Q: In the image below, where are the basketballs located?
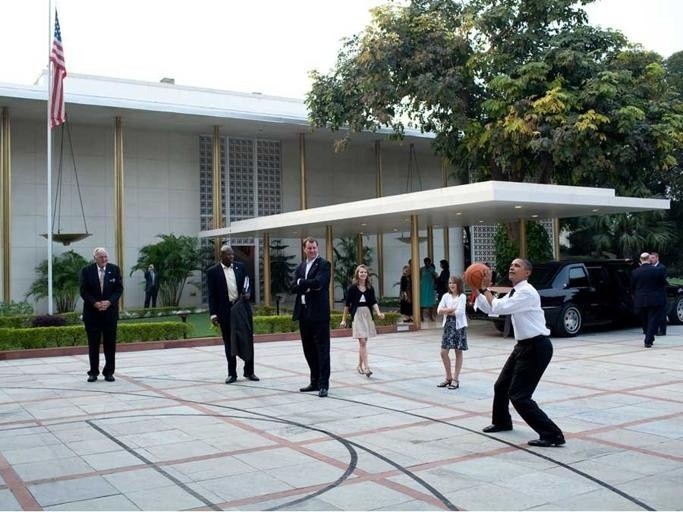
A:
[463,263,489,288]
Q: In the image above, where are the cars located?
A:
[466,259,683,336]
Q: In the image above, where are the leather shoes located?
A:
[527,434,566,447]
[86,375,98,382]
[318,388,329,397]
[300,383,319,392]
[482,423,514,433]
[224,374,238,384]
[243,372,260,382]
[105,374,115,381]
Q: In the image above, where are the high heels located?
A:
[449,379,459,389]
[437,377,452,388]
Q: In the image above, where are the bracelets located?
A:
[482,288,491,295]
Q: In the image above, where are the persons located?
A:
[631,252,666,347]
[288,239,331,397]
[144,264,159,307]
[207,245,260,383]
[79,247,124,382]
[340,265,385,377]
[470,257,566,447]
[400,258,450,323]
[436,275,468,389]
[639,252,666,336]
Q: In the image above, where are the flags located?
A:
[50,8,66,128]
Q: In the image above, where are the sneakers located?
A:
[645,341,654,348]
[356,365,374,378]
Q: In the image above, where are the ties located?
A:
[99,266,106,294]
[503,289,516,338]
